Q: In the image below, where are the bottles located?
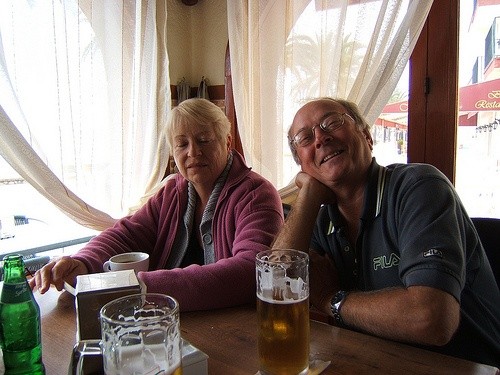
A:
[0,254,46,375]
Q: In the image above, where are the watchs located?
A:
[329,289,351,329]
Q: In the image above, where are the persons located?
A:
[28,96,286,315]
[268,96,500,369]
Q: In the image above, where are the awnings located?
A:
[375,78,499,132]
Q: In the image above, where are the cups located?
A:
[100,293,182,375]
[256,249,310,375]
[103,252,149,272]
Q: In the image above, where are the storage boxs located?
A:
[74,268,141,375]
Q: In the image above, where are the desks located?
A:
[26,271,500,375]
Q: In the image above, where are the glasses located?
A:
[289,112,355,147]
